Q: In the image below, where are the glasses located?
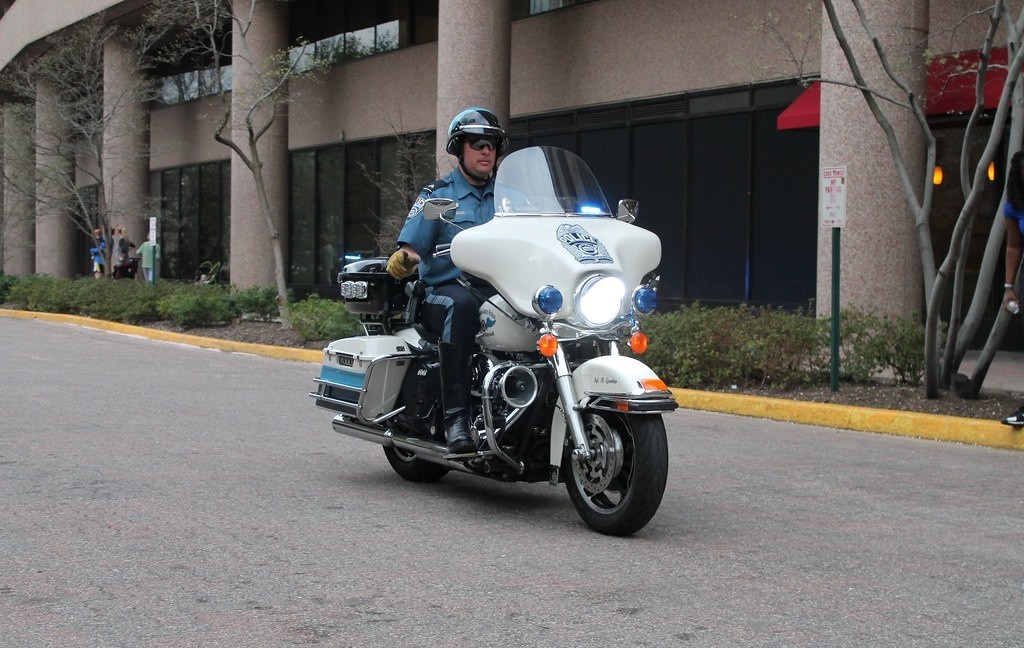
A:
[463,137,497,151]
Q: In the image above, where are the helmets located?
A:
[446,106,509,156]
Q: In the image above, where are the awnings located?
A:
[776,45,1024,130]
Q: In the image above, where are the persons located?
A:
[135,233,162,282]
[387,107,537,458]
[90,224,137,279]
[1002,152,1024,425]
[201,274,207,282]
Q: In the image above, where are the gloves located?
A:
[387,251,421,280]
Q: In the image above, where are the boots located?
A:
[437,340,478,453]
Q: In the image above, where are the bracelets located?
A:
[1005,284,1012,288]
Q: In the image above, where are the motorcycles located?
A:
[309,146,677,537]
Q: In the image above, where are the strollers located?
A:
[193,262,222,285]
[113,258,139,281]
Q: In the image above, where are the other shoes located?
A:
[1001,406,1024,425]
